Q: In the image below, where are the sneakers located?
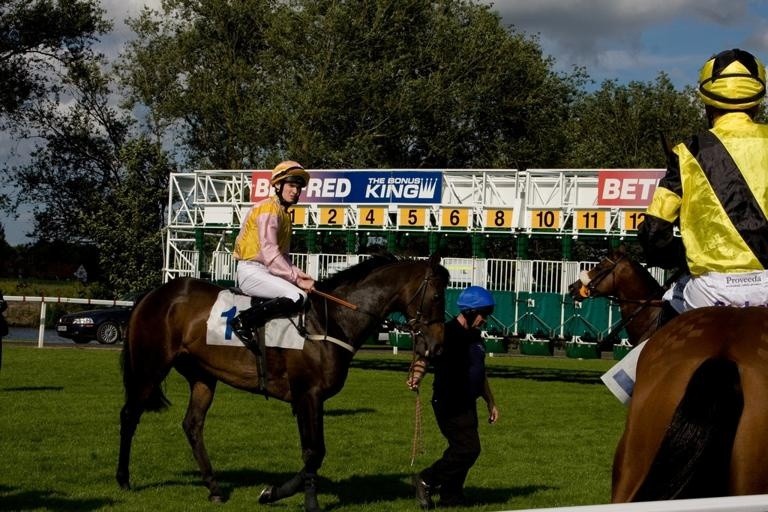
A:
[414,472,432,510]
[439,492,478,507]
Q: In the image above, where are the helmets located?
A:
[694,48,766,110]
[456,285,496,313]
[269,160,311,188]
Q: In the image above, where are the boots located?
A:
[228,297,298,356]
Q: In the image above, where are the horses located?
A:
[611,305,768,503]
[568,242,666,350]
[115,246,450,512]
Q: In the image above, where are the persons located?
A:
[405,284,498,508]
[225,159,314,353]
[635,47,767,330]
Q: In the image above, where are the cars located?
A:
[58,289,150,346]
